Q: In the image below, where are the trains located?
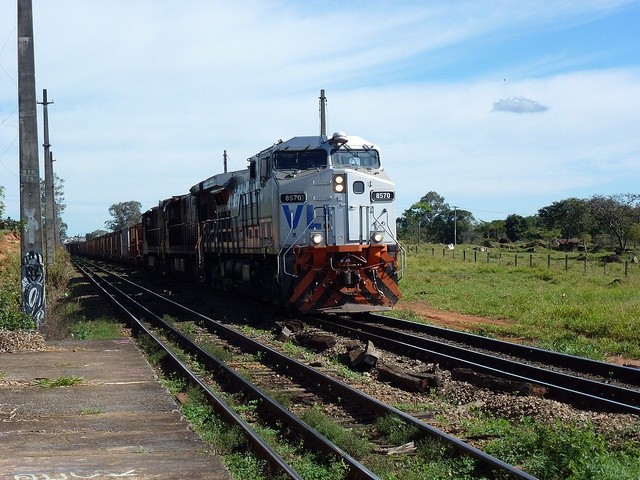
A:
[65,132,406,322]
